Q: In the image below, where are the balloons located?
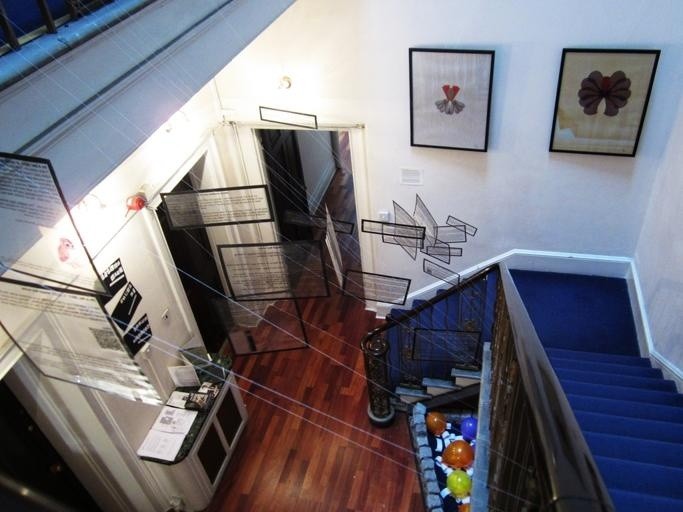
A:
[460,416,478,442]
[441,439,474,469]
[426,411,446,435]
[446,468,472,499]
[458,502,471,512]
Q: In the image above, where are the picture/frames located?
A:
[408,46,495,152]
[548,47,661,158]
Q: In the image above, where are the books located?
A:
[167,352,201,388]
[184,382,220,412]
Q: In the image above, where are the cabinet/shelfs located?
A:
[137,370,248,511]
